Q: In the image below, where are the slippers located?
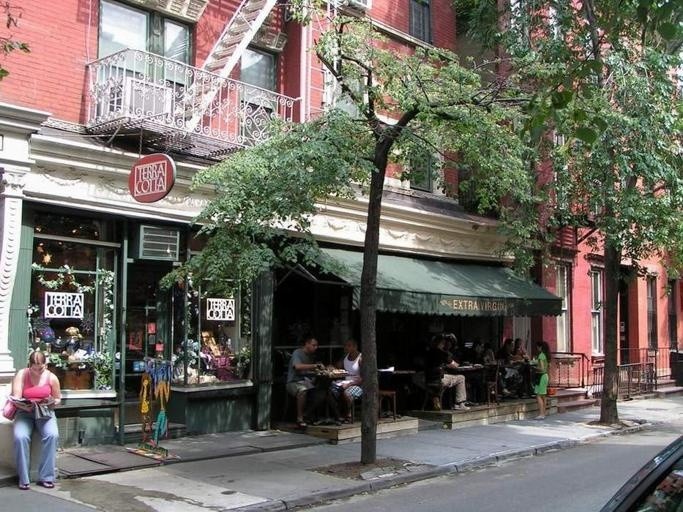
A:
[297,419,307,427]
[18,482,30,489]
[37,481,54,488]
[337,415,351,425]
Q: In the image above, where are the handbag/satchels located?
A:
[3,400,17,419]
[534,373,543,385]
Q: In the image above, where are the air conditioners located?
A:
[108,77,173,123]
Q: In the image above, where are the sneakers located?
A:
[433,397,440,411]
[454,402,471,411]
[534,415,546,420]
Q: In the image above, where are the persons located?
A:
[326,338,363,426]
[284,337,326,428]
[528,341,552,419]
[9,350,62,490]
[424,331,533,412]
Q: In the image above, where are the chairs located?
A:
[283,360,533,423]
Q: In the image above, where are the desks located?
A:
[46,362,93,389]
